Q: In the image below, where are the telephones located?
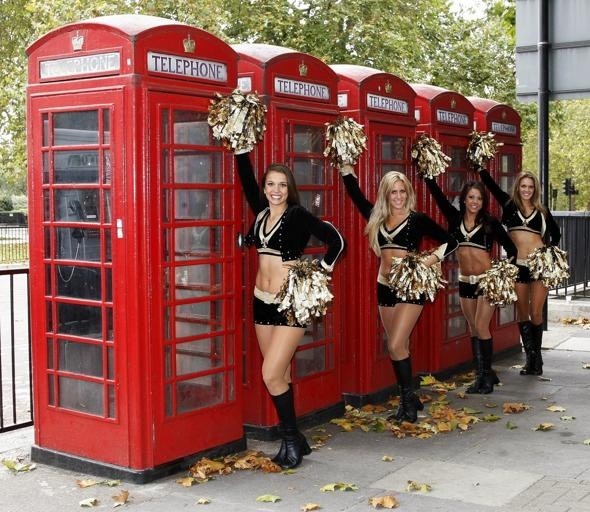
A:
[56,189,111,268]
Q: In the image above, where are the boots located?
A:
[387,353,425,426]
[517,320,545,376]
[476,337,500,394]
[465,335,483,394]
[271,383,312,470]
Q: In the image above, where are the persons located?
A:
[419,172,517,394]
[231,146,346,469]
[339,163,462,424]
[473,164,572,376]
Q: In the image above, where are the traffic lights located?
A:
[553,188,557,198]
[562,178,571,195]
